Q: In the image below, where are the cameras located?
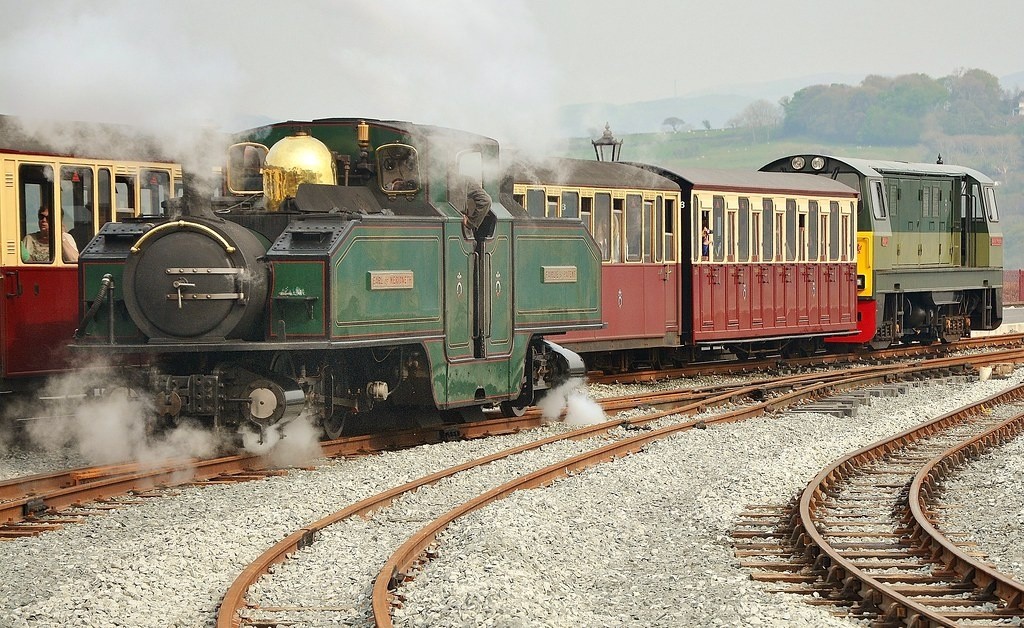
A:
[707,230,713,234]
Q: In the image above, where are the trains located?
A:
[0,118,1004,456]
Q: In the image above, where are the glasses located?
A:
[38,213,50,220]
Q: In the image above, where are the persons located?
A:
[23,203,80,264]
[594,210,631,264]
[455,165,494,233]
[699,224,716,265]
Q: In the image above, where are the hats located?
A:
[798,217,804,225]
